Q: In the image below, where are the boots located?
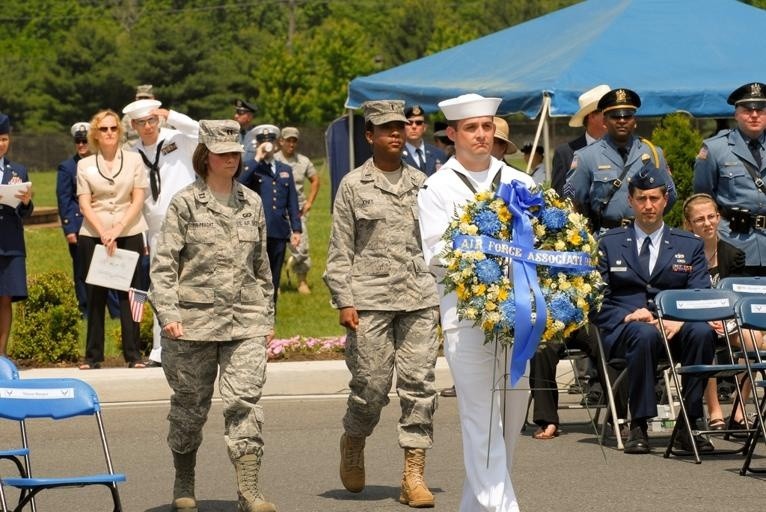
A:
[232,454,276,512]
[172,449,198,512]
[340,433,365,492]
[296,273,311,294]
[400,448,435,507]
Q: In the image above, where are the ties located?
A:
[267,162,272,171]
[639,237,650,277]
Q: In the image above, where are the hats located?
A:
[0,85,299,155]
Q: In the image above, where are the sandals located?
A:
[129,361,145,368]
[80,362,100,369]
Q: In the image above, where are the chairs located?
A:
[2,357,126,511]
[594,274,765,477]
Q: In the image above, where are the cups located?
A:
[263,143,273,153]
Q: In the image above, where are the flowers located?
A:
[443,179,605,346]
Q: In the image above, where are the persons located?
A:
[432,125,457,154]
[692,80,766,268]
[681,192,765,432]
[56,122,121,321]
[121,84,173,149]
[552,84,608,198]
[519,145,546,187]
[76,107,151,369]
[277,128,321,296]
[147,119,278,512]
[231,100,256,139]
[533,318,635,441]
[0,111,34,353]
[418,91,537,511]
[122,99,201,367]
[566,90,677,229]
[589,167,717,456]
[321,96,439,507]
[241,125,302,306]
[490,117,524,173]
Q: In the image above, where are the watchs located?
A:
[397,105,446,179]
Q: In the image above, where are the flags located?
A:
[127,287,148,323]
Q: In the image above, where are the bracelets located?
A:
[117,221,125,227]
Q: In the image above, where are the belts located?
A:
[718,204,766,231]
[600,218,634,229]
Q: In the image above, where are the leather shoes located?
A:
[441,384,456,397]
[568,375,757,454]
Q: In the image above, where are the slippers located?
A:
[532,424,558,439]
